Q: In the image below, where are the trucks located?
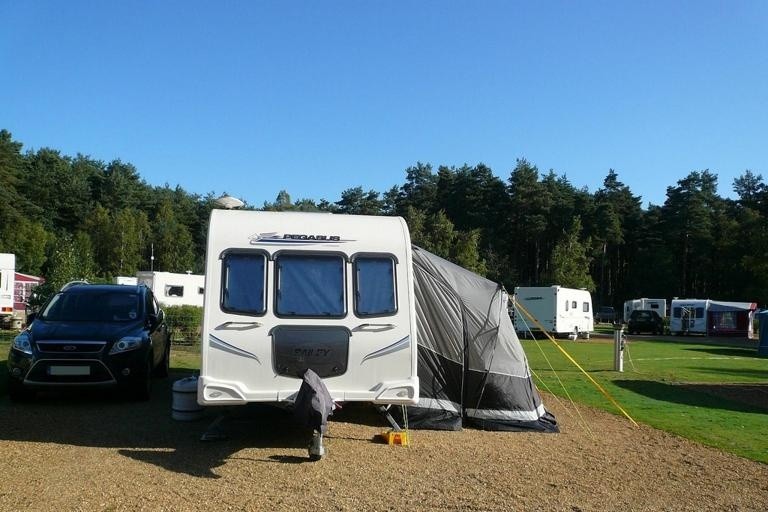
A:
[599,307,618,322]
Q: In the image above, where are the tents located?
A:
[375,243,562,435]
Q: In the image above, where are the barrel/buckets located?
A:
[10,317,23,329]
[173,376,206,421]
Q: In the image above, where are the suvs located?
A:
[627,310,663,336]
[7,280,172,400]
